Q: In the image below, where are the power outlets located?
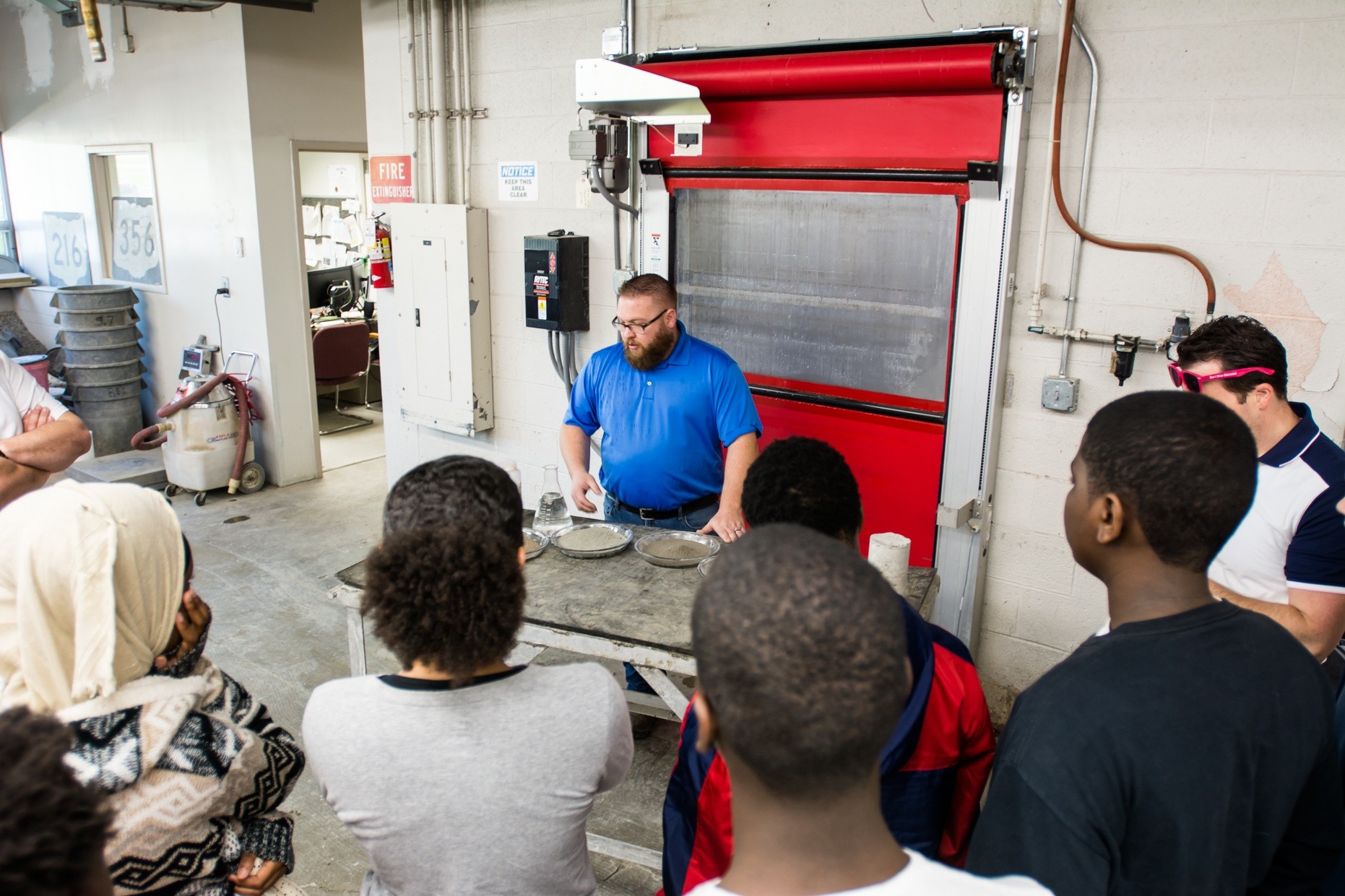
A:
[223,278,230,298]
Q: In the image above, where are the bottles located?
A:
[505,459,522,500]
[532,464,573,538]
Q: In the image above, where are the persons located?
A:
[1,349,306,896]
[300,457,631,895]
[1178,315,1345,702]
[561,273,762,542]
[964,390,1345,896]
[682,526,1057,896]
[661,435,990,896]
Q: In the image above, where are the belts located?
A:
[607,490,719,520]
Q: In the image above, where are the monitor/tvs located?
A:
[309,258,370,320]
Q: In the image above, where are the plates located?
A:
[634,531,720,567]
[523,528,550,561]
[550,523,634,559]
[695,556,718,577]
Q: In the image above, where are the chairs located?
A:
[314,322,373,435]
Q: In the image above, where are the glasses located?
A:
[612,308,667,334]
[1167,361,1276,394]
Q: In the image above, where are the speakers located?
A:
[363,299,374,320]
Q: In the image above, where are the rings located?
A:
[735,529,741,533]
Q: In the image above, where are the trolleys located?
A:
[163,350,267,507]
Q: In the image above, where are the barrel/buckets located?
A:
[49,285,148,458]
[11,354,50,391]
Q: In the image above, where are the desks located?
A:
[309,309,381,404]
[326,508,943,870]
